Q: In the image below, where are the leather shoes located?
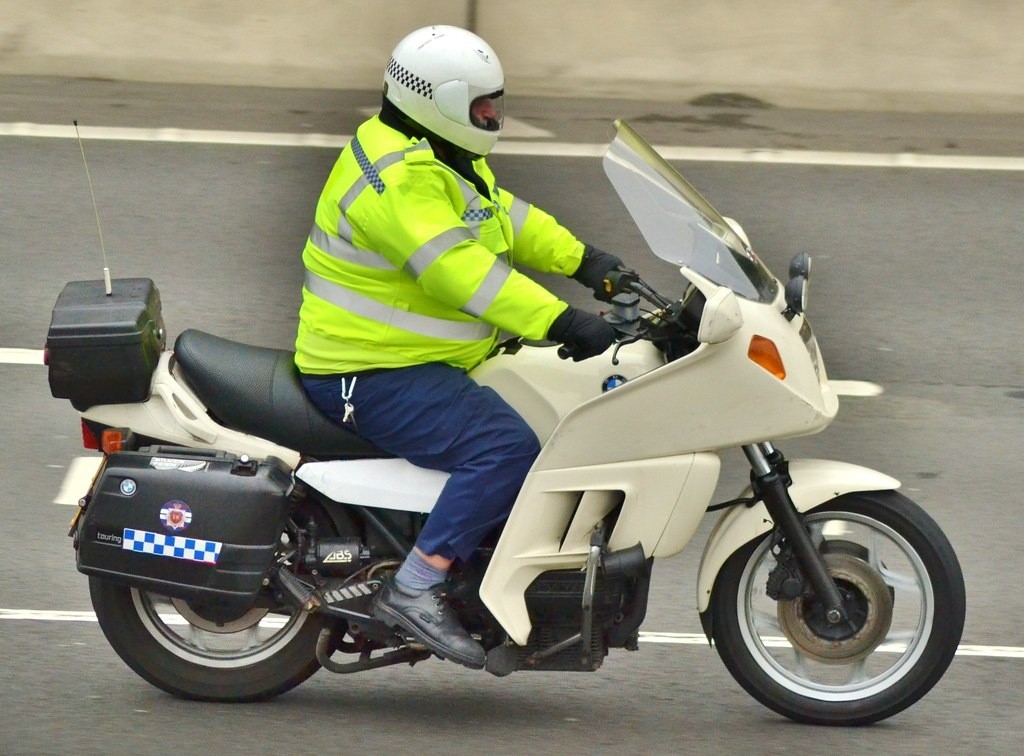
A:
[367,577,488,669]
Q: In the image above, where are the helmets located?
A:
[382,24,509,162]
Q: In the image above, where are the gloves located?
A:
[577,244,628,303]
[547,305,618,362]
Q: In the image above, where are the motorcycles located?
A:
[42,118,968,726]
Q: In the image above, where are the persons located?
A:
[295,24,628,669]
